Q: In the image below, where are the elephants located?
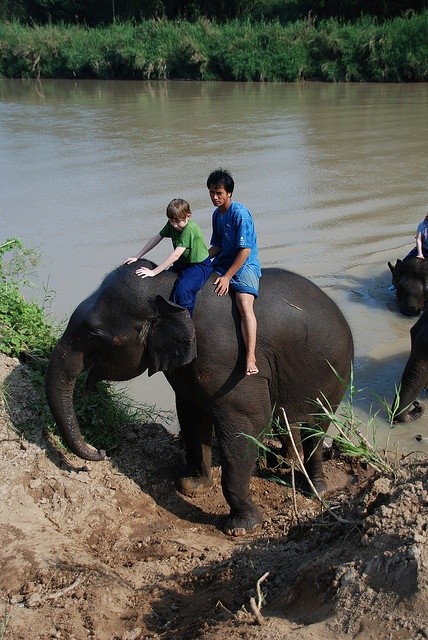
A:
[387,245,427,317]
[390,297,427,422]
[44,257,353,537]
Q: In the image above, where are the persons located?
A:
[415,212,427,261]
[121,198,214,310]
[207,166,262,375]
